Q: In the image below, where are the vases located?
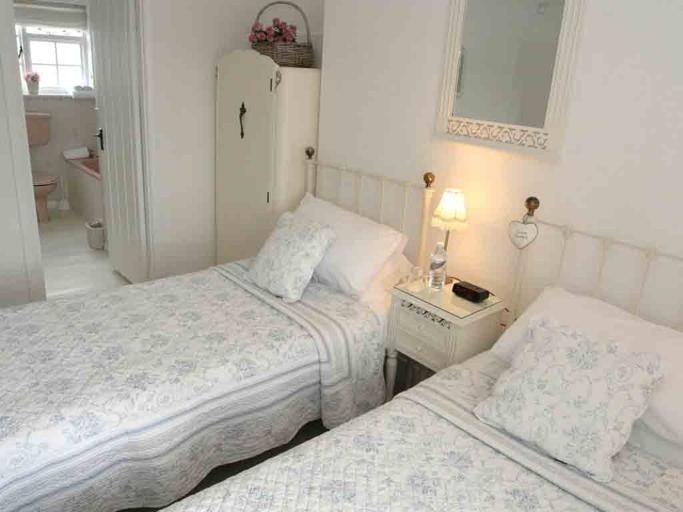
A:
[26,81,38,95]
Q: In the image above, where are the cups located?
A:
[407,265,427,293]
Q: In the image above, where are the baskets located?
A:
[252,2,314,65]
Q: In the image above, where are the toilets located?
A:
[24,112,57,224]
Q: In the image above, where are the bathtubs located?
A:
[66,157,100,179]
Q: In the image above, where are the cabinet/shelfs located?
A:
[212,48,318,264]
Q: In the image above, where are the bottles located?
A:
[430,242,448,291]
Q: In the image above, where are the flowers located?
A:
[23,72,40,87]
[248,17,297,49]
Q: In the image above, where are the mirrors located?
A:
[434,1,584,155]
